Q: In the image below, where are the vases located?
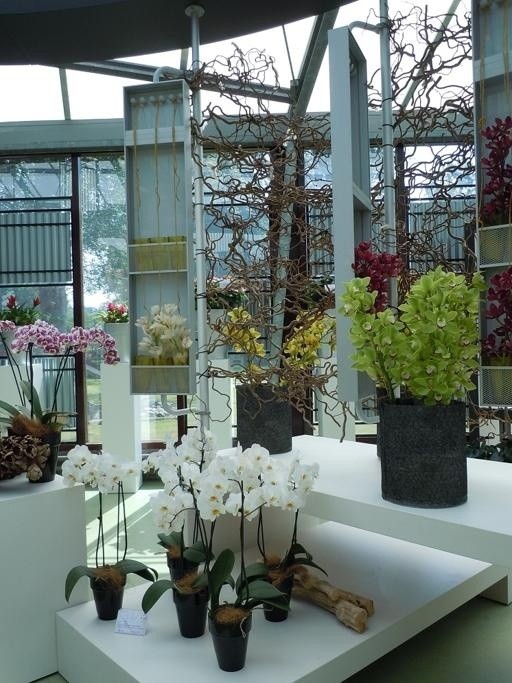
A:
[236,383,292,454]
[91,573,292,672]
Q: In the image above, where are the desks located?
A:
[53,433,512,682]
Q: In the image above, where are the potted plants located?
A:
[338,264,487,509]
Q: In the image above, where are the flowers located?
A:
[219,306,338,386]
[0,295,121,481]
[140,432,329,611]
[61,445,159,602]
[134,304,194,365]
